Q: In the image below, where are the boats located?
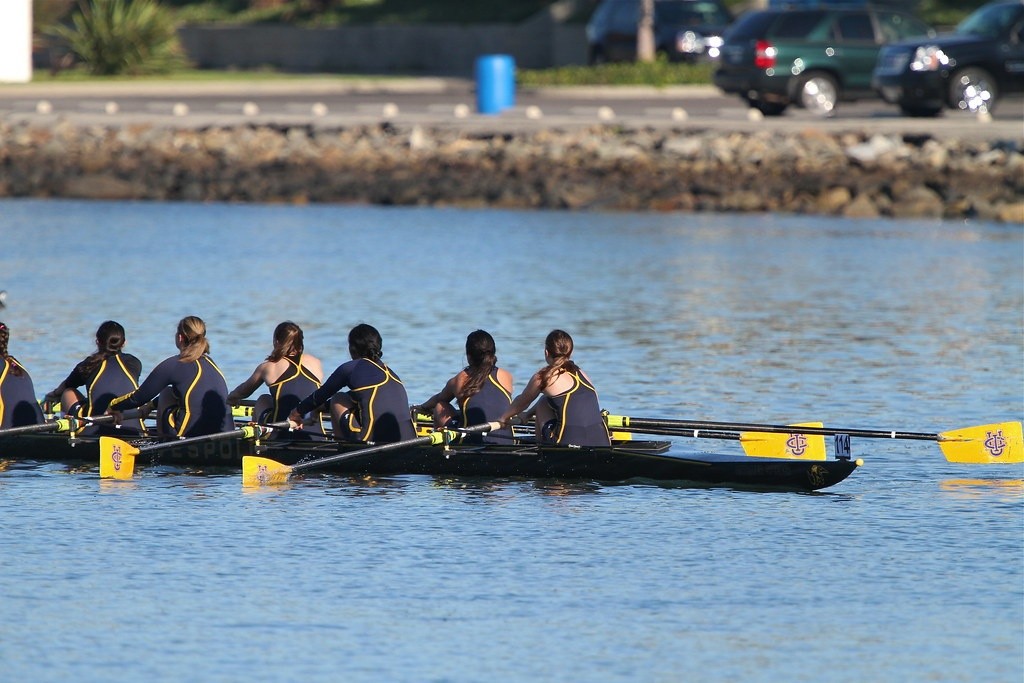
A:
[0,404,866,492]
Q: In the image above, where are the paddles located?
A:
[0,387,1024,496]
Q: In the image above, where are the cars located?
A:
[710,0,943,121]
[869,0,1024,116]
[582,1,739,66]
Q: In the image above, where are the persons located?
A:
[285,323,418,446]
[407,328,519,445]
[493,329,611,448]
[225,320,328,443]
[0,322,48,432]
[38,320,148,439]
[102,316,235,444]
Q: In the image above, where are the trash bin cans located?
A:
[473,54,515,113]
